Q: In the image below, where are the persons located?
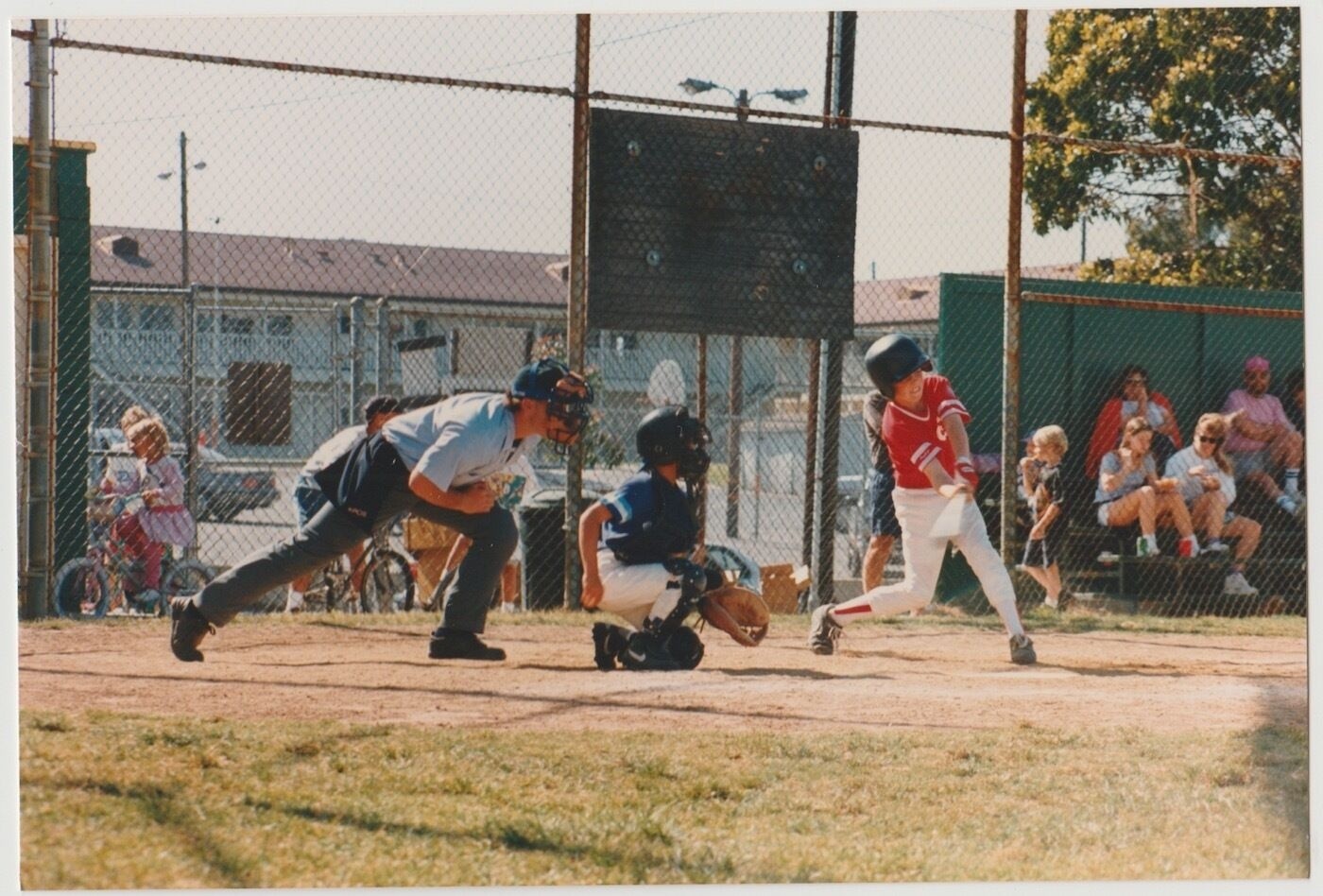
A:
[96,405,196,615]
[808,335,1035,664]
[171,358,593,665]
[1018,425,1079,612]
[576,406,728,668]
[439,453,542,612]
[287,396,404,613]
[862,389,929,616]
[1085,357,1307,594]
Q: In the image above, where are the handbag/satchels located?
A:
[313,436,400,536]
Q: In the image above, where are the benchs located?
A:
[985,472,1307,616]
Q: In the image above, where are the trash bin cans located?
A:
[514,486,604,613]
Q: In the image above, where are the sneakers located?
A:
[809,604,844,655]
[592,624,623,671]
[429,631,507,661]
[1009,634,1036,664]
[169,596,216,662]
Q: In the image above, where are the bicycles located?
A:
[303,509,415,613]
[49,491,216,621]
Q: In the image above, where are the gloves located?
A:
[954,458,978,494]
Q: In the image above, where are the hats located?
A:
[1020,430,1037,442]
[1245,355,1270,371]
[512,356,571,402]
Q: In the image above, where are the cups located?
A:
[1179,540,1191,558]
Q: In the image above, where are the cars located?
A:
[167,442,277,529]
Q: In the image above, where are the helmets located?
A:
[865,333,933,400]
[636,405,704,472]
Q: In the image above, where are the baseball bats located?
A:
[930,492,968,544]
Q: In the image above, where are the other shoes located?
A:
[1209,539,1228,553]
[1199,536,1220,556]
[1040,603,1053,610]
[1148,551,1174,560]
[106,600,155,618]
[1058,590,1074,611]
[1285,486,1302,503]
[1180,536,1210,557]
[1224,574,1258,596]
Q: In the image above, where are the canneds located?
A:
[1136,537,1149,557]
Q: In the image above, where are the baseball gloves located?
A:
[697,585,770,647]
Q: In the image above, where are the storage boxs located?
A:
[406,515,461,551]
[726,564,799,614]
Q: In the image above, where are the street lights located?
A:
[156,128,207,556]
[677,77,809,540]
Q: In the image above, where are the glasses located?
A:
[1248,373,1269,380]
[1196,432,1218,444]
